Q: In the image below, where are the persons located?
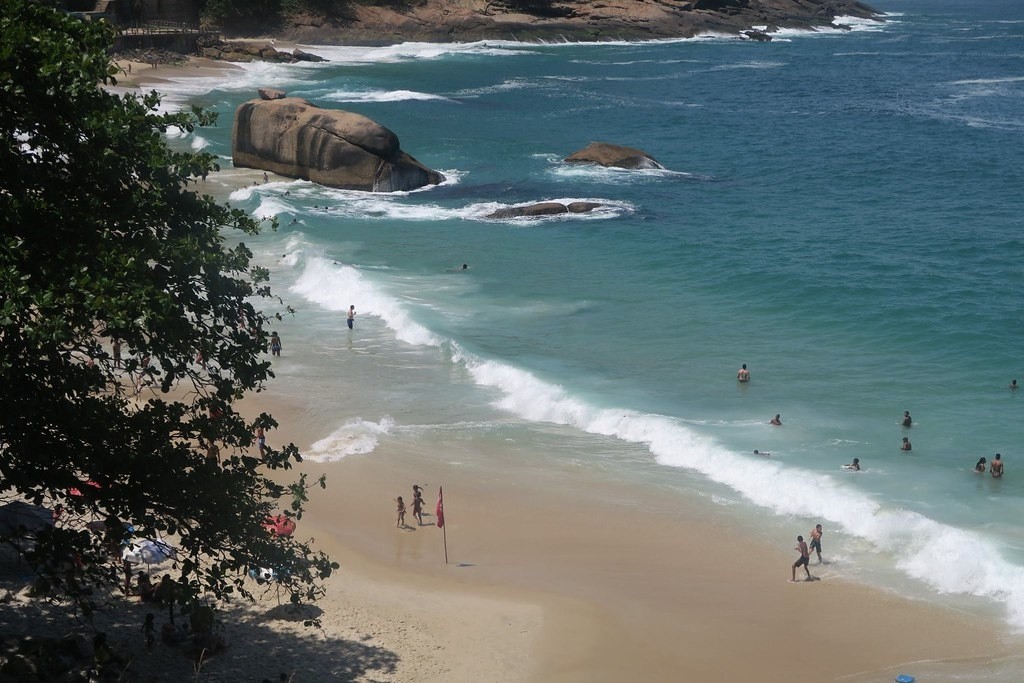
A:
[902,410,912,428]
[807,524,823,564]
[1009,379,1019,391]
[753,449,770,456]
[410,485,425,525]
[393,496,407,527]
[989,453,1004,479]
[346,305,356,330]
[736,363,750,383]
[768,414,782,425]
[242,171,470,272]
[976,457,986,473]
[900,437,912,451]
[83,304,305,649]
[849,458,860,472]
[787,535,812,583]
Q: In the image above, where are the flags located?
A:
[436,486,444,528]
